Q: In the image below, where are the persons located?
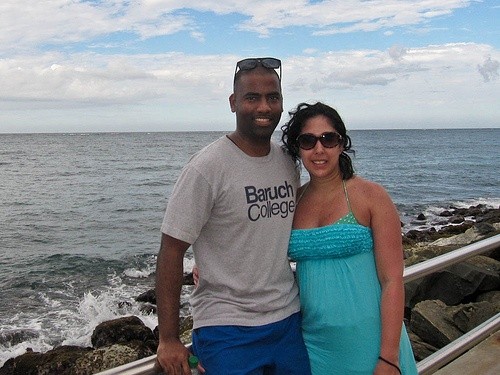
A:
[192,101,418,375]
[157,62,311,375]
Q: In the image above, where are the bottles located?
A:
[181,355,201,375]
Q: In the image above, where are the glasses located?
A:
[233,58,282,87]
[294,131,345,150]
